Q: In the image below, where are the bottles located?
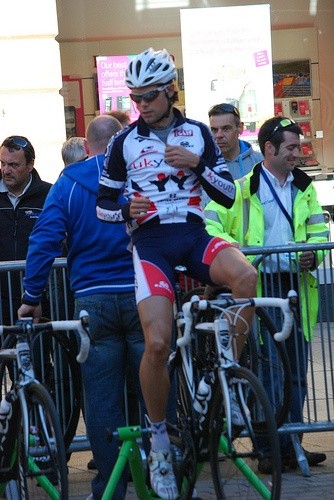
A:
[192,375,211,414]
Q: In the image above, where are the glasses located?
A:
[129,84,170,103]
[222,104,240,119]
[268,118,296,141]
[5,137,35,159]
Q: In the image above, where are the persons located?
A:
[0,135,80,488]
[204,102,264,183]
[16,113,181,500]
[95,48,262,500]
[60,135,90,169]
[202,114,333,473]
[104,111,131,128]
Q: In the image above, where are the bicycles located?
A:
[144,261,299,500]
[0,310,92,497]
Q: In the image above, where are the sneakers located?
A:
[146,449,180,500]
[194,376,251,426]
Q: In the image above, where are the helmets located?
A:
[124,47,176,89]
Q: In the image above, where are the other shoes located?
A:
[281,442,326,468]
[254,445,285,474]
